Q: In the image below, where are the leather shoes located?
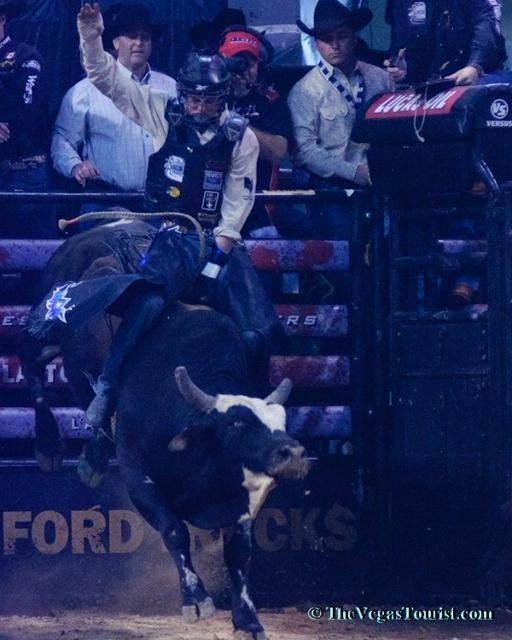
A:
[83,374,121,431]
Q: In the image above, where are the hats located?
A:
[101,6,166,51]
[295,0,373,37]
[220,30,265,60]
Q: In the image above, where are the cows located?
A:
[11,204,313,639]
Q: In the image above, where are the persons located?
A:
[0,0,510,306]
[76,2,271,442]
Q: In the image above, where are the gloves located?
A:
[191,243,229,304]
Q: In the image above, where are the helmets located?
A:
[175,47,232,124]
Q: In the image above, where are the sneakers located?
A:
[441,291,474,320]
